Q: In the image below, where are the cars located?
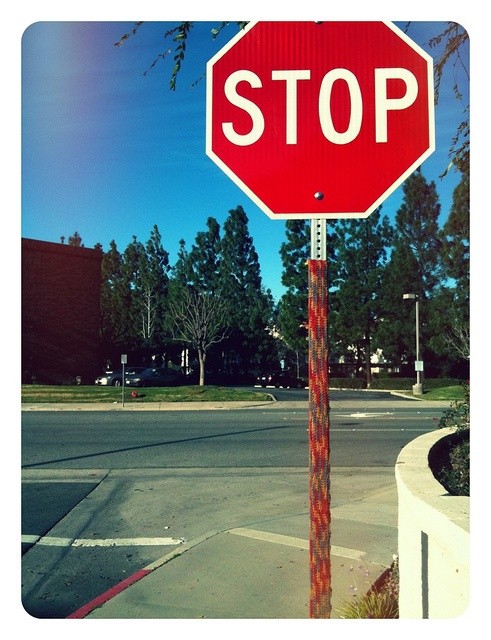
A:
[126,368,186,387]
[257,371,305,389]
[95,367,146,386]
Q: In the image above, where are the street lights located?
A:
[403,294,421,383]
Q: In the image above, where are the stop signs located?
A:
[205,21,435,220]
